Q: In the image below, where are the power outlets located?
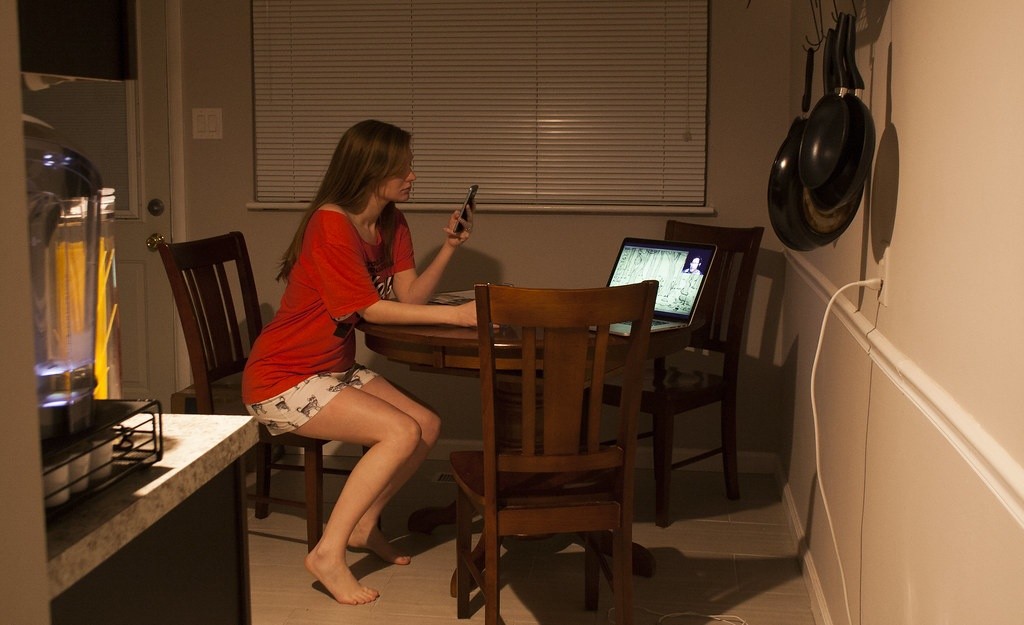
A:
[874,241,890,306]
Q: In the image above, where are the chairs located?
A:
[156,231,382,558]
[445,279,660,625]
[582,220,765,528]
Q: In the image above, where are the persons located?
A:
[242,119,500,604]
[684,255,703,275]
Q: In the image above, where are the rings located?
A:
[465,225,472,231]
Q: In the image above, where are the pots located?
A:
[766,12,875,250]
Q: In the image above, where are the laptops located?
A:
[589,238,718,337]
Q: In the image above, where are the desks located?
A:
[353,289,706,599]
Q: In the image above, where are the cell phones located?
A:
[453,184,479,233]
